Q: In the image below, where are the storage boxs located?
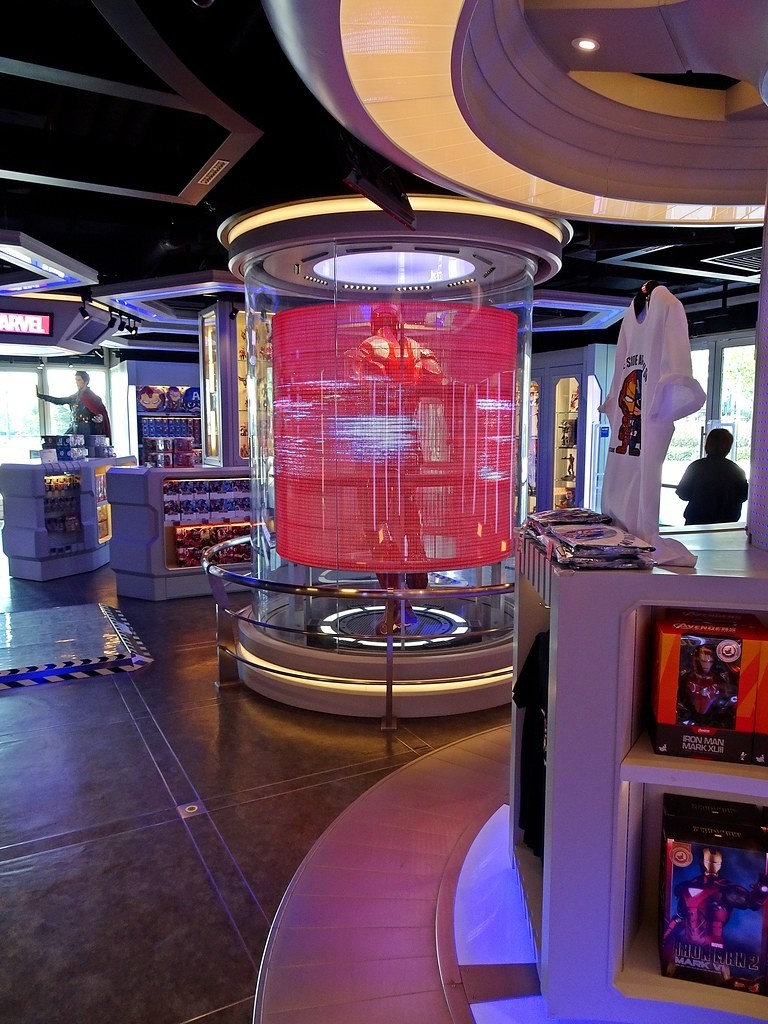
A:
[41,435,115,459]
[142,417,201,468]
[163,479,253,567]
[650,610,768,768]
[659,793,768,997]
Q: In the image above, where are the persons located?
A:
[346,302,471,636]
[36,371,112,445]
[562,454,574,475]
[676,429,749,526]
[677,645,739,729]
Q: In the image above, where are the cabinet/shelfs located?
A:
[0,455,138,581]
[106,466,252,601]
[554,410,579,483]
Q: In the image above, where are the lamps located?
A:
[78,296,93,320]
[108,306,142,336]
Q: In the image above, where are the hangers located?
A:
[632,278,670,314]
